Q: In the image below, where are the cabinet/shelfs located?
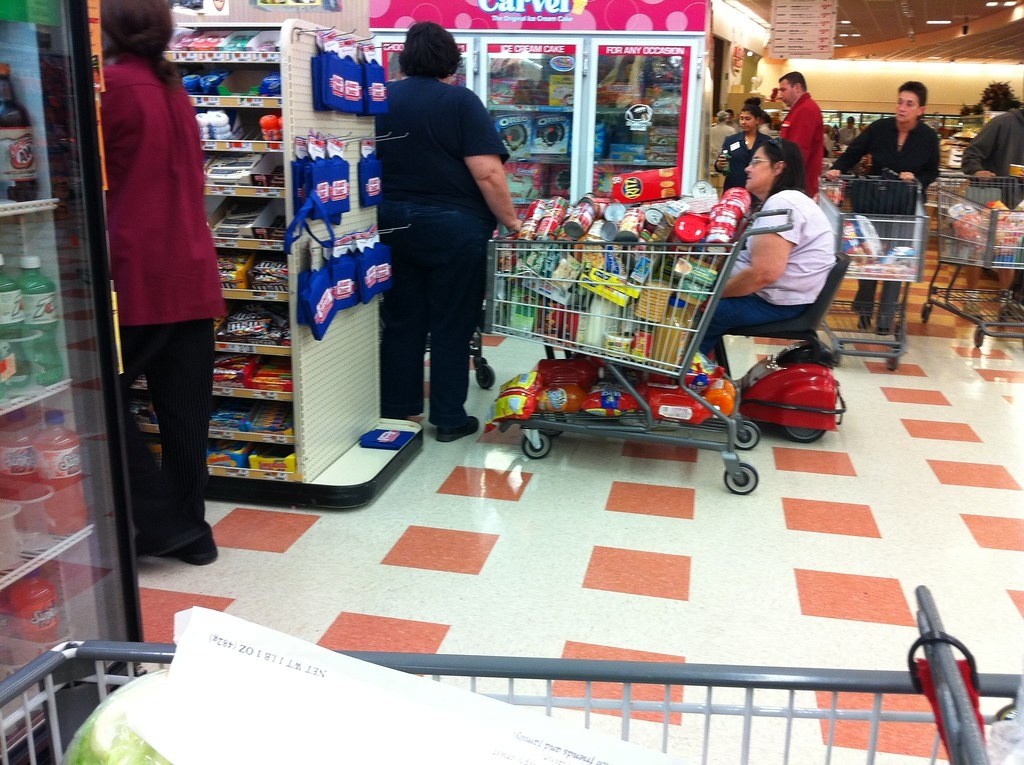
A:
[164,17,424,512]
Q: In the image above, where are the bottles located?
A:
[648,297,691,372]
[0,253,65,400]
[0,62,39,201]
[981,202,1003,246]
[722,150,731,176]
[534,358,604,414]
[0,568,61,648]
[648,376,735,423]
[0,408,88,537]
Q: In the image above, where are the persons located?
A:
[378,21,523,443]
[697,138,837,378]
[710,109,739,163]
[778,71,825,203]
[828,81,939,334]
[837,115,860,144]
[94,0,227,566]
[961,107,1024,304]
[714,97,774,196]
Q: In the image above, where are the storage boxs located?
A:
[648,212,675,251]
[167,25,297,478]
[589,269,642,299]
[489,77,682,219]
[521,278,577,306]
[630,255,662,284]
[578,273,629,308]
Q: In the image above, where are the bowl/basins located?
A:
[604,331,635,358]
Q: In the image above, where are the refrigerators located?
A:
[0,1,142,765]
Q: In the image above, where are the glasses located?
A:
[748,159,773,166]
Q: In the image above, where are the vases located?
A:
[990,102,1009,111]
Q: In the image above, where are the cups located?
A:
[1,483,56,572]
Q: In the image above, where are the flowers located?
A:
[980,82,1020,107]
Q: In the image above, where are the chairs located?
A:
[713,250,851,379]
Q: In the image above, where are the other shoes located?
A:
[858,315,870,329]
[877,327,890,335]
[438,415,478,441]
[135,530,218,564]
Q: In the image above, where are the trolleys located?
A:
[471,206,795,498]
[922,172,1024,347]
[820,166,930,371]
[0,580,1024,765]
